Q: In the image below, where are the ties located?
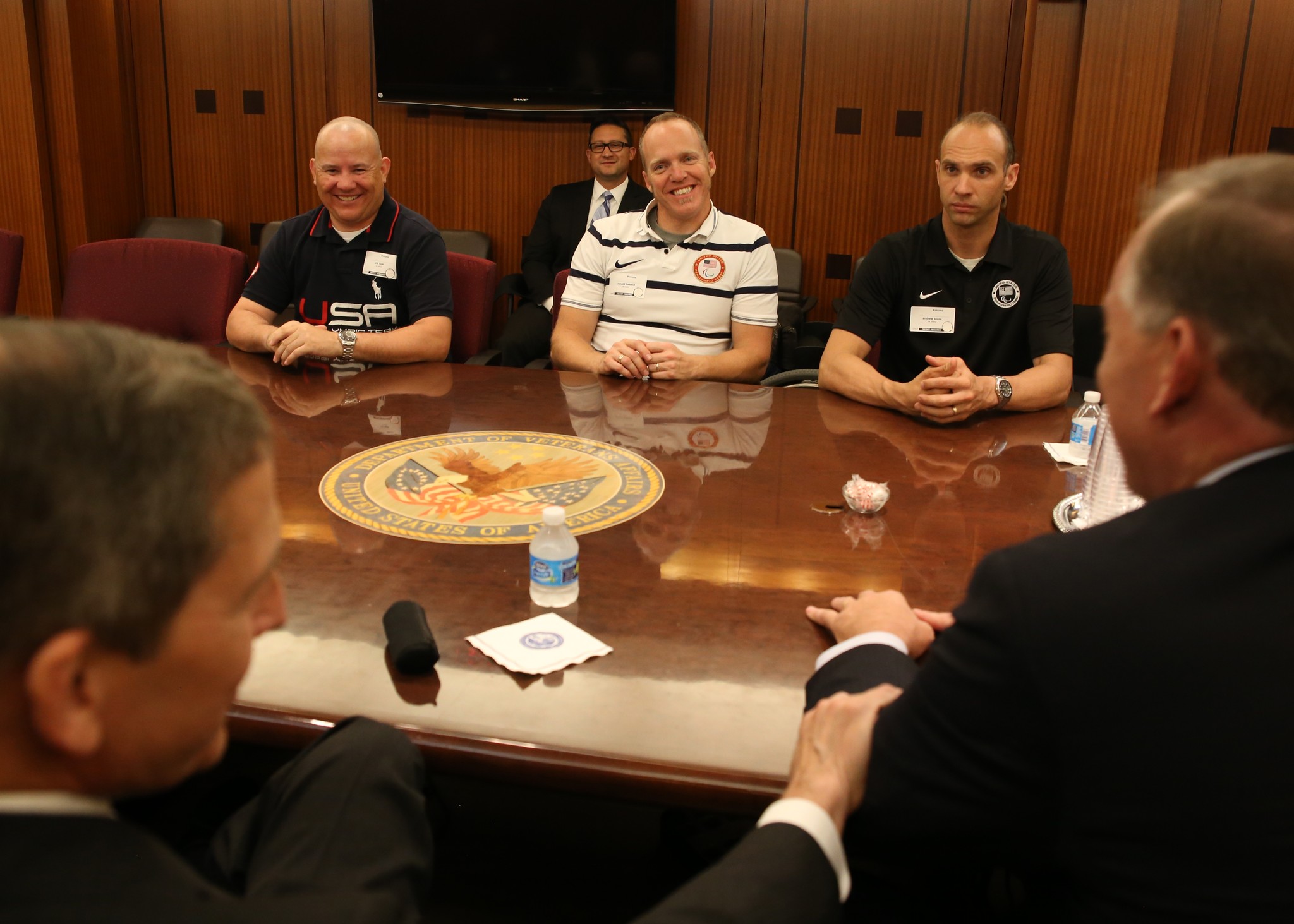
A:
[591,192,613,226]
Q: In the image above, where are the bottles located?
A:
[529,506,581,608]
[1067,391,1101,461]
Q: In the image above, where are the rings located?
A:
[618,354,625,363]
[951,405,958,415]
[656,363,659,371]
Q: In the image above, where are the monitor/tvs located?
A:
[370,0,679,122]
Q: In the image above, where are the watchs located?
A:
[985,375,1012,410]
[338,330,357,364]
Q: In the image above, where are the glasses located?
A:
[589,141,630,152]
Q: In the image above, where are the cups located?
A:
[1080,404,1147,529]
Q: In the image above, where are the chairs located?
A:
[134,215,225,244]
[60,238,246,348]
[1070,303,1105,391]
[444,251,504,368]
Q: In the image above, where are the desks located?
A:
[167,347,1141,815]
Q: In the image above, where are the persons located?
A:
[1,319,906,924]
[226,117,454,364]
[807,152,1294,923]
[497,115,654,367]
[549,111,779,383]
[816,111,1074,424]
